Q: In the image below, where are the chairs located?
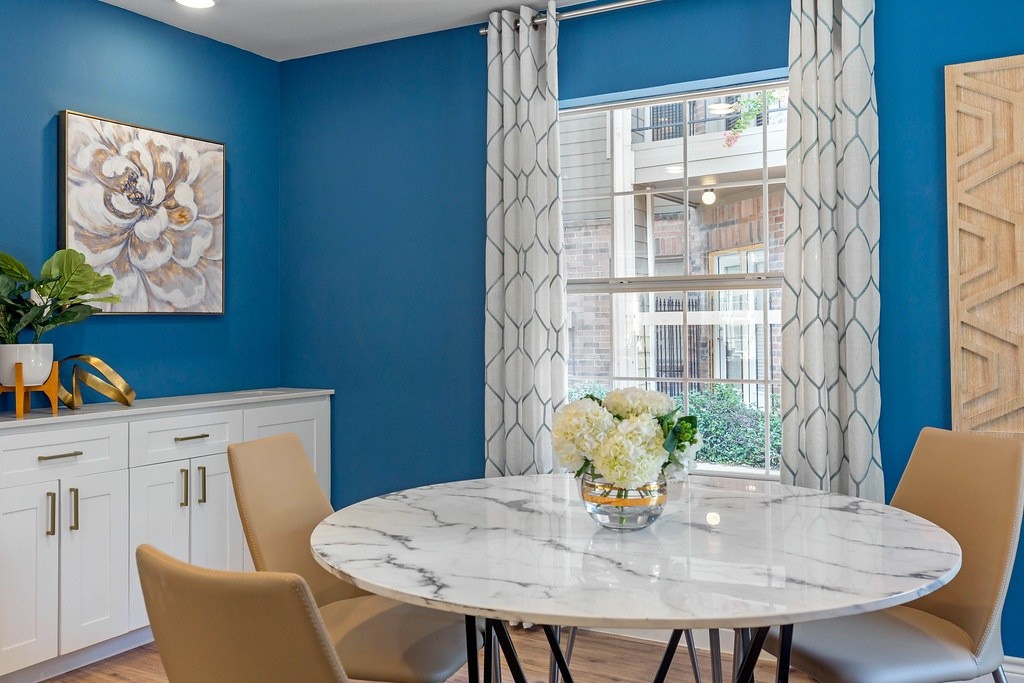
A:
[229,434,504,683]
[134,544,348,683]
[739,426,1024,683]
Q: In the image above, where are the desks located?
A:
[311,471,962,683]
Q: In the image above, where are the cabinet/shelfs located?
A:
[0,386,336,683]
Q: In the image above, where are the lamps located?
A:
[702,189,717,205]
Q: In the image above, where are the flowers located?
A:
[552,386,704,509]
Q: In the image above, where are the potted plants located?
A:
[0,248,123,388]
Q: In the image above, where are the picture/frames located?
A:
[57,109,226,318]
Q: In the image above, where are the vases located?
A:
[579,467,668,533]
